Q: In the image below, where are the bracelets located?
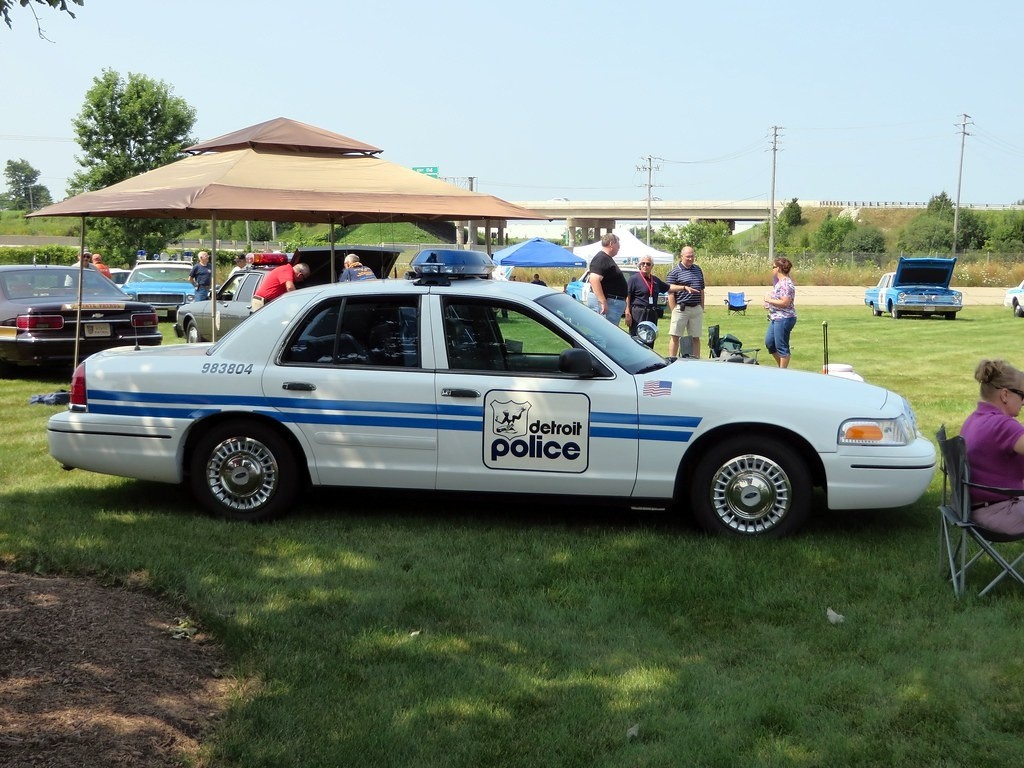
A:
[684,286,685,290]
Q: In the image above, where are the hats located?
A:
[78,246,92,255]
[233,253,246,262]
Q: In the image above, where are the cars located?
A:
[174,246,407,344]
[865,256,962,320]
[1003,280,1024,317]
[48,249,935,539]
[565,264,667,318]
[106,250,196,321]
[0,252,162,380]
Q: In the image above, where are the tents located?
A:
[574,228,673,270]
[25,116,566,373]
[494,237,586,268]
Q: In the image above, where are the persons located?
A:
[92,254,111,277]
[763,258,797,367]
[531,274,546,286]
[188,252,212,302]
[228,254,251,286]
[66,247,96,286]
[338,254,376,282]
[625,256,700,349]
[587,233,629,326]
[254,263,310,304]
[961,359,1024,535]
[666,246,706,358]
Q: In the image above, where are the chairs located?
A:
[936,423,1024,603]
[707,324,760,364]
[447,318,487,370]
[723,291,752,316]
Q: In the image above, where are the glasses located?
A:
[773,266,778,269]
[299,270,306,280]
[83,255,91,259]
[995,386,1024,400]
[641,262,651,266]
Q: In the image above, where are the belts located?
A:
[685,303,697,307]
[970,499,1006,510]
[635,305,656,308]
[253,296,268,302]
[608,295,625,300]
